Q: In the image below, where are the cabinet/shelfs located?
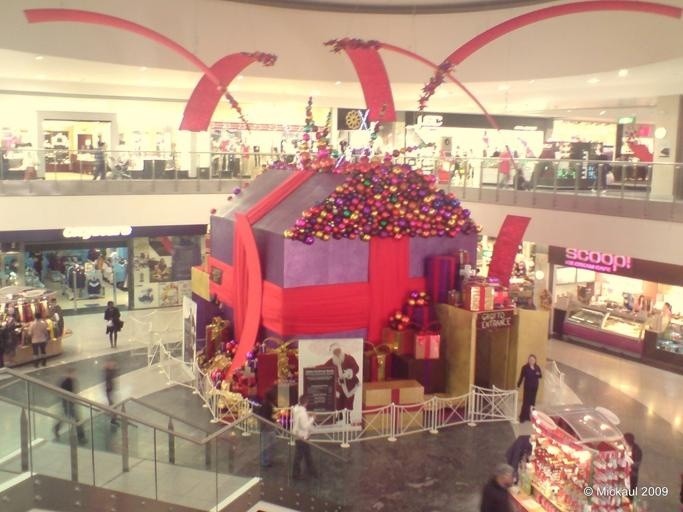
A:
[562,306,648,360]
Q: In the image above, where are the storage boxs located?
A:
[415,330,440,360]
[382,328,415,356]
[426,250,508,312]
[363,379,424,431]
[363,350,392,382]
[406,305,432,330]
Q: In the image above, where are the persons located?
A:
[515,354,542,424]
[498,144,512,189]
[31,251,44,283]
[508,434,532,470]
[27,312,48,368]
[103,301,124,349]
[621,432,642,503]
[51,298,62,317]
[479,463,517,512]
[290,394,318,478]
[256,386,283,467]
[92,141,107,181]
[629,295,644,317]
[52,364,89,445]
[103,357,121,425]
[323,343,360,425]
[657,302,672,340]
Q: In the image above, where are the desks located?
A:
[508,487,545,512]
[3,337,60,367]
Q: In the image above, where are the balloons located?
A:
[211,338,255,382]
[387,288,432,331]
[209,94,484,245]
